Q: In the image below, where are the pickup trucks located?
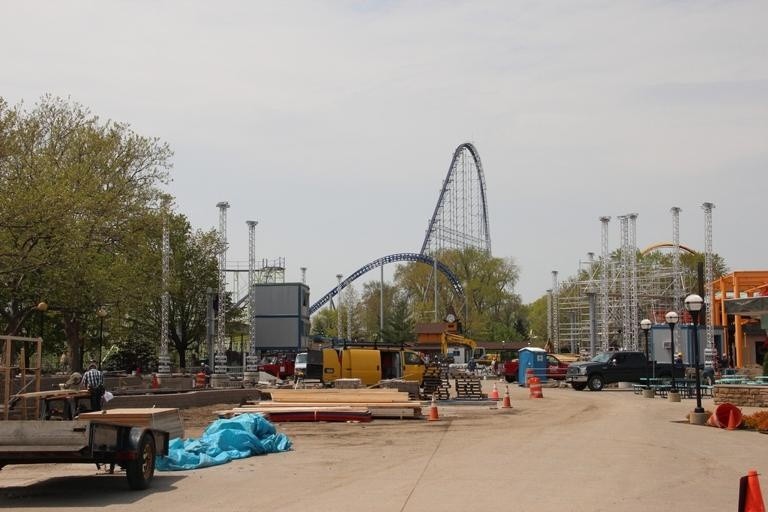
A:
[504,355,569,383]
[566,352,687,391]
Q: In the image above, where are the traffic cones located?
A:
[492,383,513,408]
[427,395,440,421]
[743,469,765,512]
[705,403,743,430]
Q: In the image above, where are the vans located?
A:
[322,347,434,387]
[294,353,307,378]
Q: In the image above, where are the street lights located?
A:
[640,312,679,393]
[684,294,704,413]
[38,302,47,363]
[96,308,107,370]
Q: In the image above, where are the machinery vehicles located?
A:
[441,330,500,369]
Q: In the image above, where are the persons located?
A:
[82,365,104,411]
[490,357,496,375]
[675,353,683,364]
[701,367,714,386]
[466,356,475,373]
[721,353,729,369]
[200,362,213,390]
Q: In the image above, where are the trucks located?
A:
[247,356,294,380]
